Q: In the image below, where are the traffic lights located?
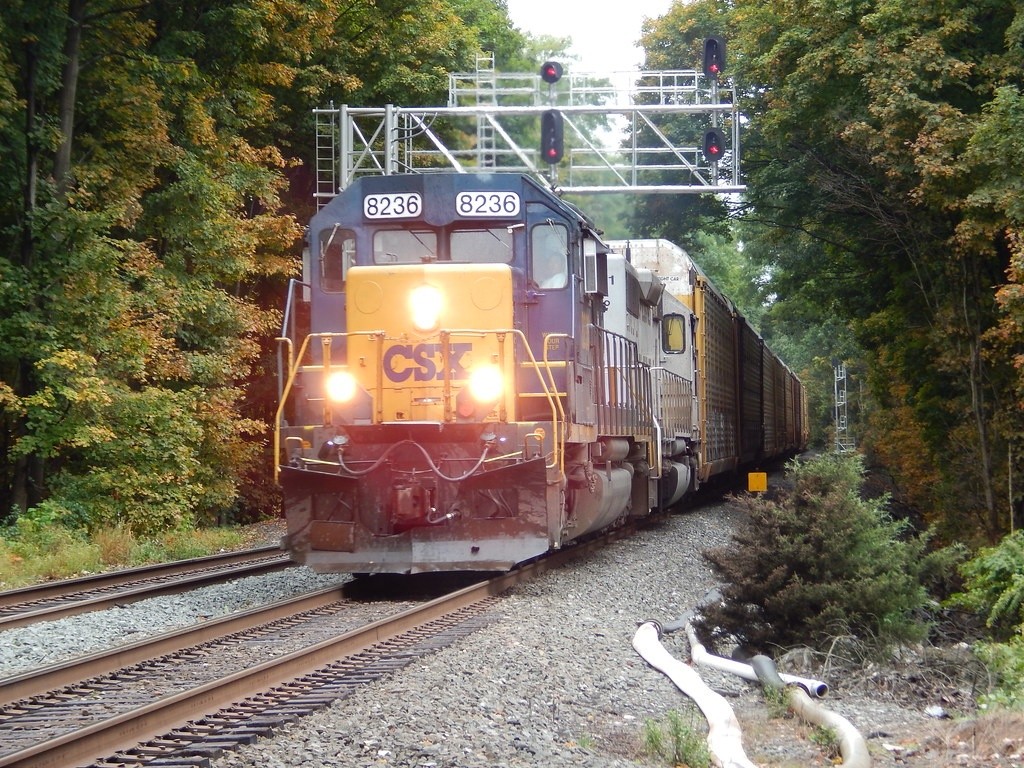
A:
[540,60,564,83]
[701,127,725,161]
[701,34,727,78]
[540,109,564,164]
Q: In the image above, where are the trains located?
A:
[270,168,810,575]
